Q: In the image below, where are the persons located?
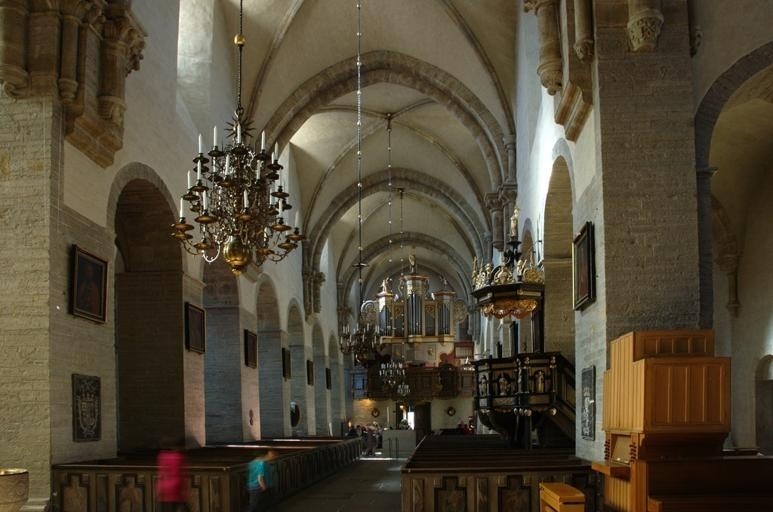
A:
[245,446,278,512]
[156,436,195,511]
[347,419,382,457]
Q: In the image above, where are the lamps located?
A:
[170,0,305,276]
[469,209,544,320]
[339,0,410,397]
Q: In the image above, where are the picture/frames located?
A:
[70,244,107,324]
[185,301,205,354]
[572,222,595,311]
[244,329,257,369]
[582,366,595,441]
[72,374,100,441]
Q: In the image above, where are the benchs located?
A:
[51,436,363,512]
[400,434,604,512]
